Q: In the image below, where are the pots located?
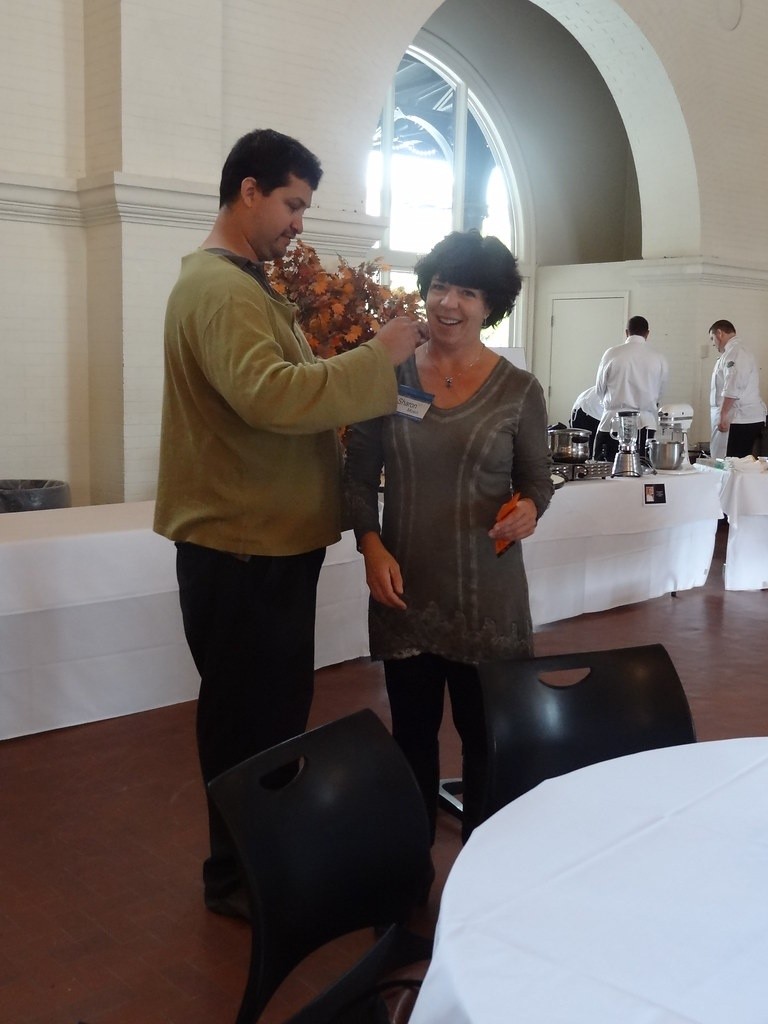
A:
[547,427,592,463]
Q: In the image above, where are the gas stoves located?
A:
[550,459,613,481]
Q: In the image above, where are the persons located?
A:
[152,129,421,920]
[708,319,768,459]
[569,315,669,466]
[342,228,555,903]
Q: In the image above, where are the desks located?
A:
[0,456,731,740]
[413,734,768,1024]
[700,456,768,592]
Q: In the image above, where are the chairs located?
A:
[439,640,698,842]
[205,704,435,1024]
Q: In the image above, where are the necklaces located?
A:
[425,339,486,389]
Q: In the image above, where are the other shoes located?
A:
[215,892,250,920]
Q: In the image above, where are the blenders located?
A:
[646,403,701,474]
[610,410,643,477]
